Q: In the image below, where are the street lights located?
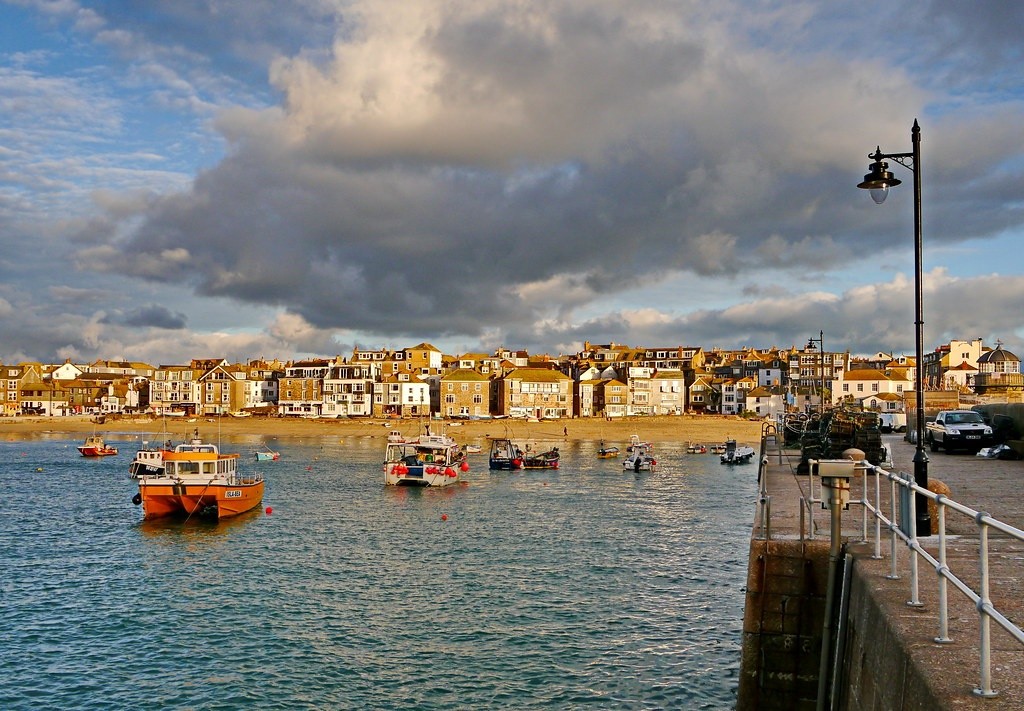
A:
[806,330,825,414]
[856,117,931,536]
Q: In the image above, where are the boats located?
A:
[255,452,280,461]
[163,411,186,417]
[598,436,657,471]
[321,414,339,418]
[77,435,118,456]
[488,438,560,470]
[128,441,165,479]
[383,430,482,486]
[230,411,251,417]
[432,413,558,428]
[687,439,755,465]
[139,428,264,519]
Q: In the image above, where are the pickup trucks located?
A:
[926,409,993,454]
